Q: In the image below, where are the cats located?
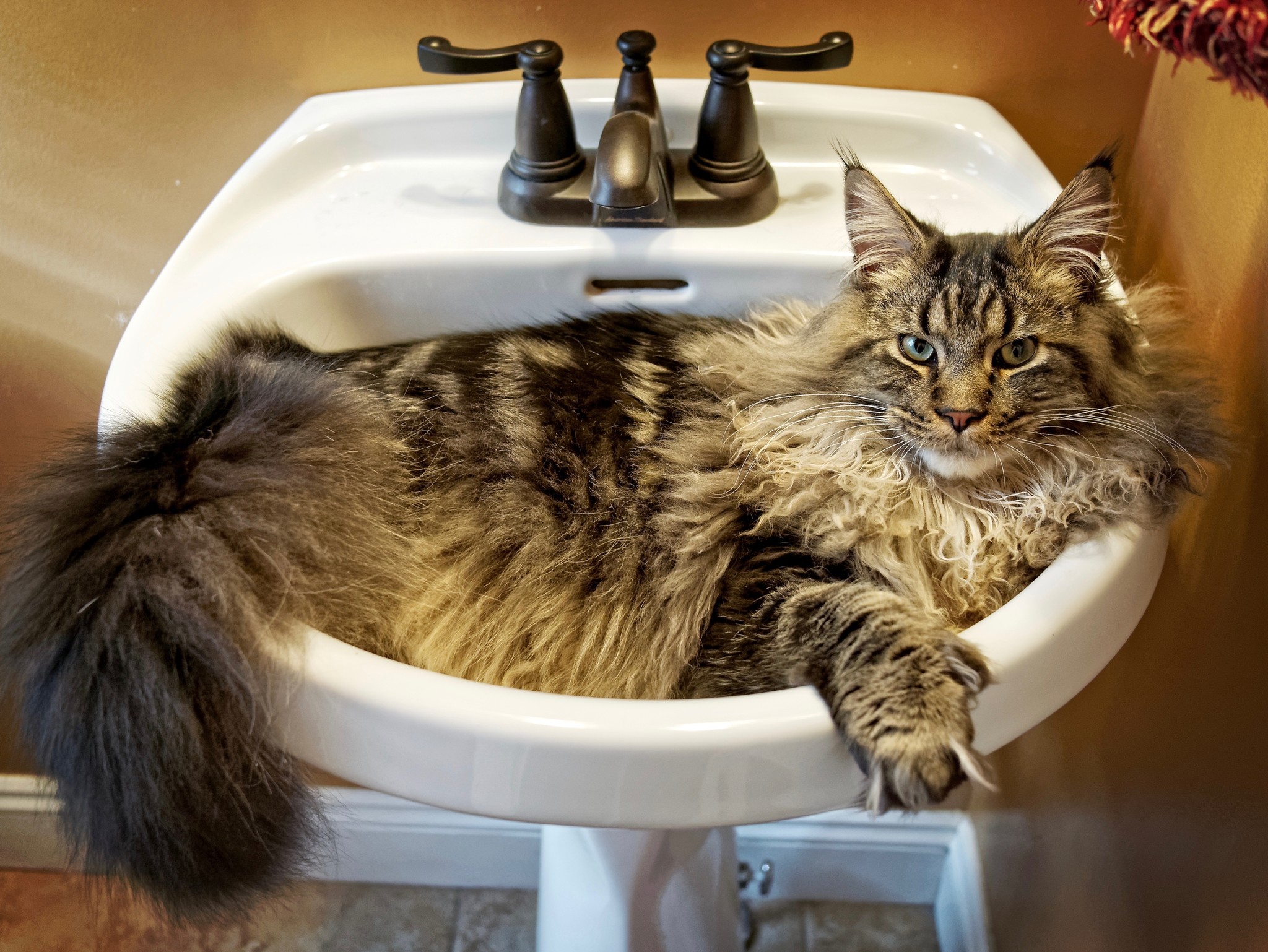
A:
[1,138,1238,938]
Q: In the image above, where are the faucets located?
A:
[413,17,856,231]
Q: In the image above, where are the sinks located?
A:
[98,71,1172,829]
